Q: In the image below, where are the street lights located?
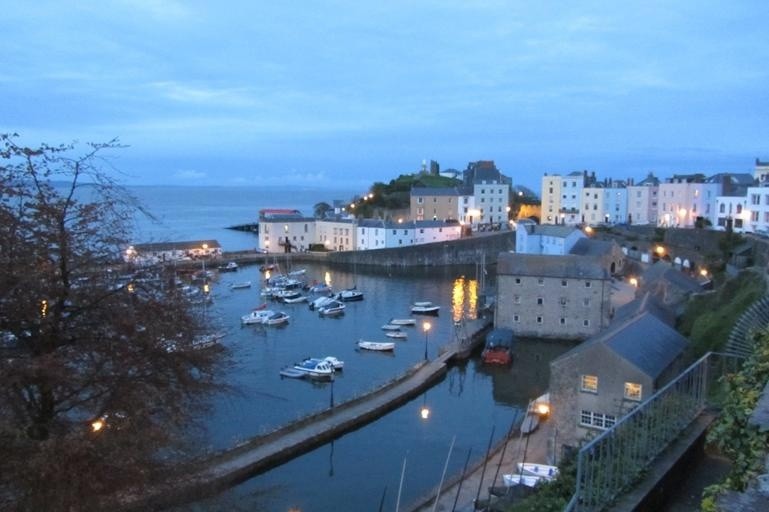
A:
[424,323,430,360]
[330,373,335,407]
[422,391,428,418]
[329,442,334,477]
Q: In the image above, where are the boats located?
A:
[478,463,559,512]
[217,262,362,325]
[176,269,213,304]
[411,302,440,314]
[279,356,344,381]
[521,400,540,433]
[359,319,416,351]
[481,329,512,365]
